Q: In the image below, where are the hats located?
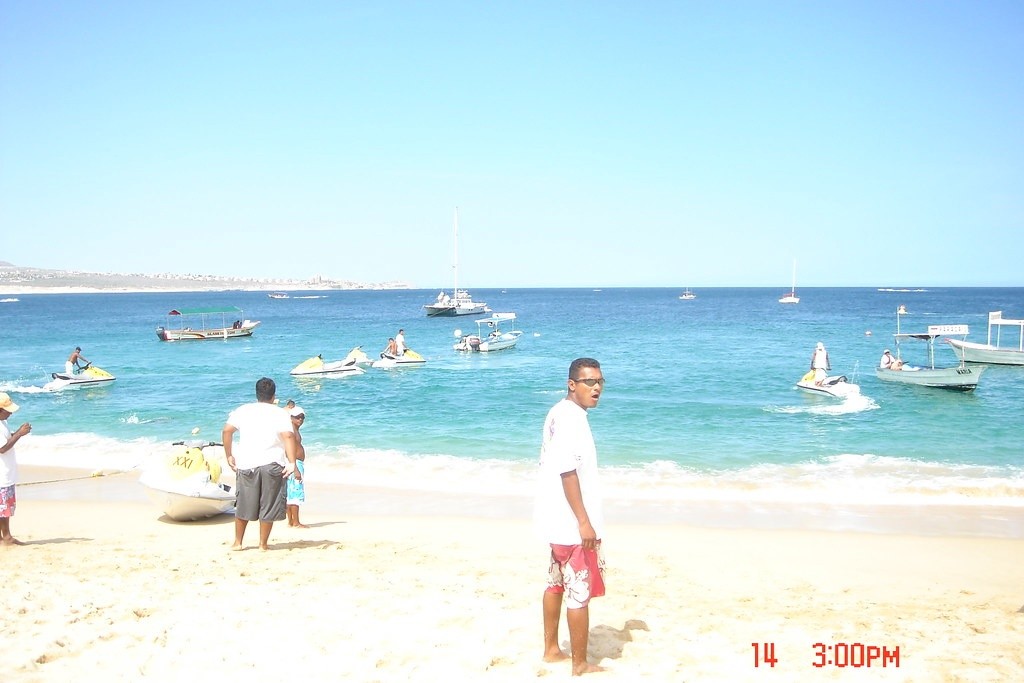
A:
[291,407,305,417]
[0,392,19,412]
[884,349,890,354]
[76,347,82,351]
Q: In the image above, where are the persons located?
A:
[288,407,311,529]
[881,349,911,371]
[223,377,295,551]
[65,347,91,375]
[281,401,295,411]
[0,392,32,544]
[233,321,242,329]
[540,358,608,675]
[384,338,398,356]
[396,329,407,356]
[811,342,831,382]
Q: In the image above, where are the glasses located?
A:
[574,378,605,386]
[292,416,305,419]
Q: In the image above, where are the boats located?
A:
[479,329,522,352]
[872,360,986,394]
[373,350,426,367]
[810,342,832,371]
[945,337,1023,365]
[778,295,800,303]
[128,469,235,521]
[425,204,490,316]
[288,352,361,377]
[49,363,117,386]
[678,295,696,299]
[795,372,848,397]
[155,321,258,341]
